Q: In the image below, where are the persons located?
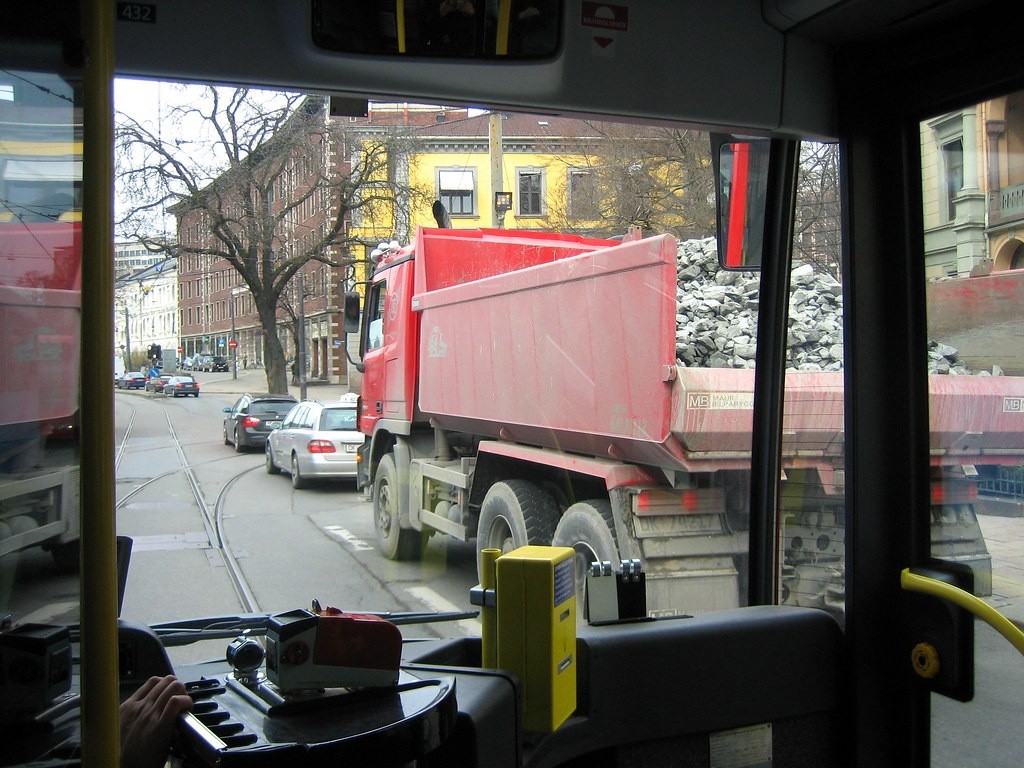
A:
[116,672,193,768]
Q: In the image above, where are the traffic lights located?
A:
[148,345,162,360]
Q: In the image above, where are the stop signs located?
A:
[229,340,237,349]
[178,347,183,353]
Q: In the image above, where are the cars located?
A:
[163,376,199,398]
[223,391,300,453]
[115,371,173,393]
[265,400,365,489]
[183,353,229,372]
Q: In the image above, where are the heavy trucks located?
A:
[343,226,1023,625]
[1,70,84,573]
